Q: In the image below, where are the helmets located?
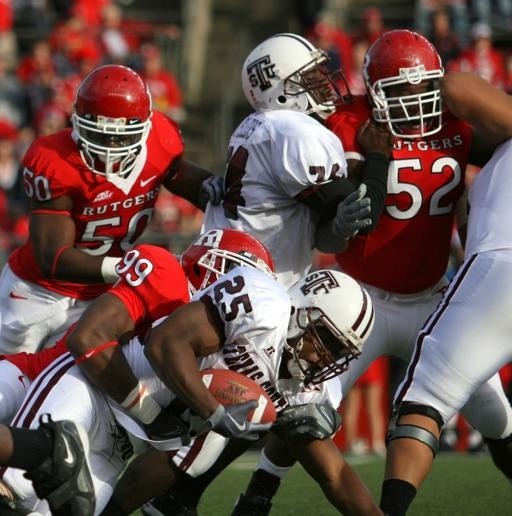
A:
[70,62,156,177]
[239,31,355,122]
[281,267,376,386]
[180,225,275,293]
[359,26,449,141]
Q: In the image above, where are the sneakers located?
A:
[21,408,97,516]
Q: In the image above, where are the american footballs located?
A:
[198,370,278,424]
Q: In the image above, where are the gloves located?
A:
[354,117,396,161]
[142,403,194,445]
[204,396,278,443]
[330,182,375,241]
[270,398,344,443]
[196,172,228,211]
